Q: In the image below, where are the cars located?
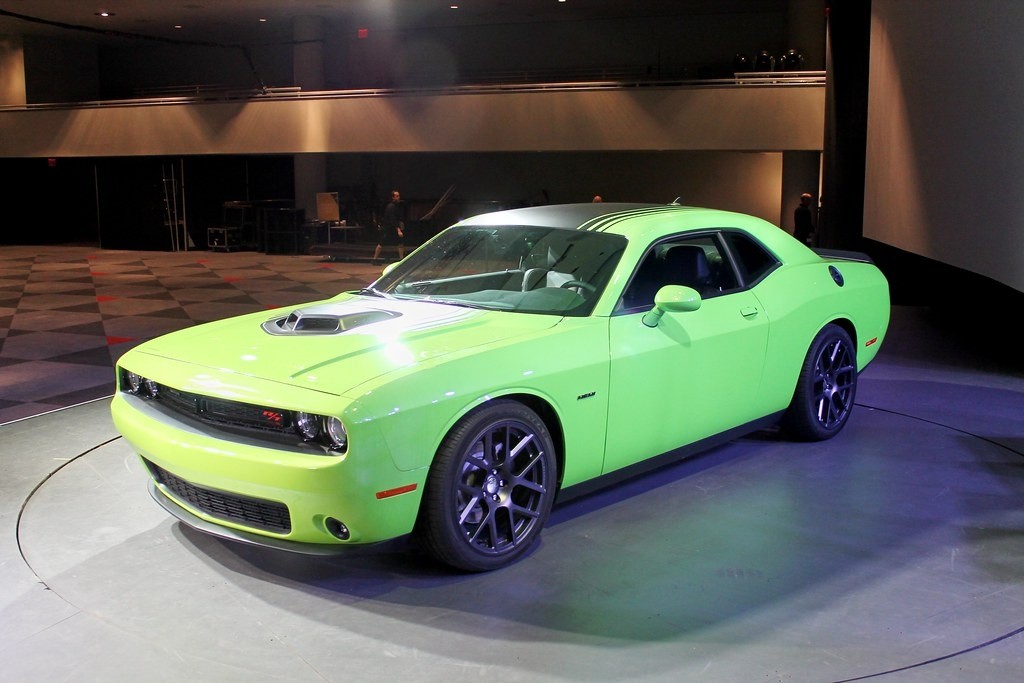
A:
[108,199,893,571]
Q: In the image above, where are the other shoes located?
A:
[371,257,377,266]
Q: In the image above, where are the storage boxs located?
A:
[255,200,305,255]
[207,200,251,248]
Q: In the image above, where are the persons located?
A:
[370,191,404,266]
[793,193,813,248]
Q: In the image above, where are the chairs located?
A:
[664,246,720,298]
[522,244,586,297]
